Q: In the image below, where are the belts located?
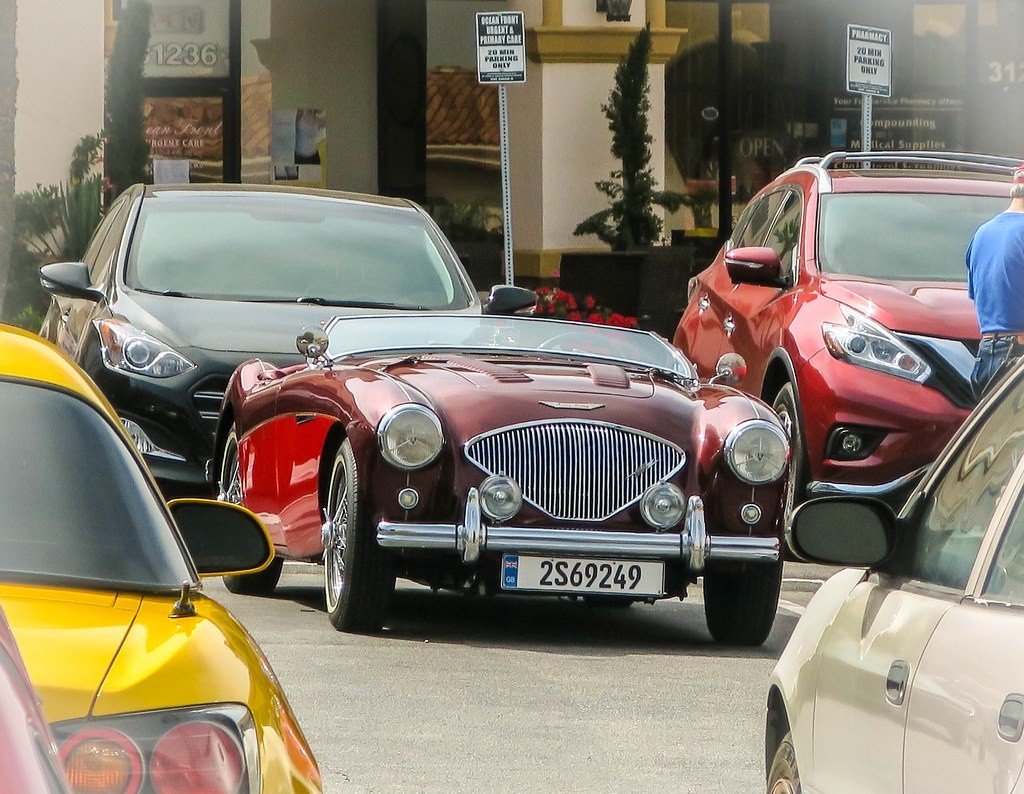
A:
[982,331,1024,338]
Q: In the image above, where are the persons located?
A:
[965,167,1024,400]
[294,109,326,165]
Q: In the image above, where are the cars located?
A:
[762,350,1024,794]
[42,181,541,539]
[0,326,324,787]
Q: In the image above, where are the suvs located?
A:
[673,151,1024,539]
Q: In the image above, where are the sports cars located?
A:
[213,318,796,649]
